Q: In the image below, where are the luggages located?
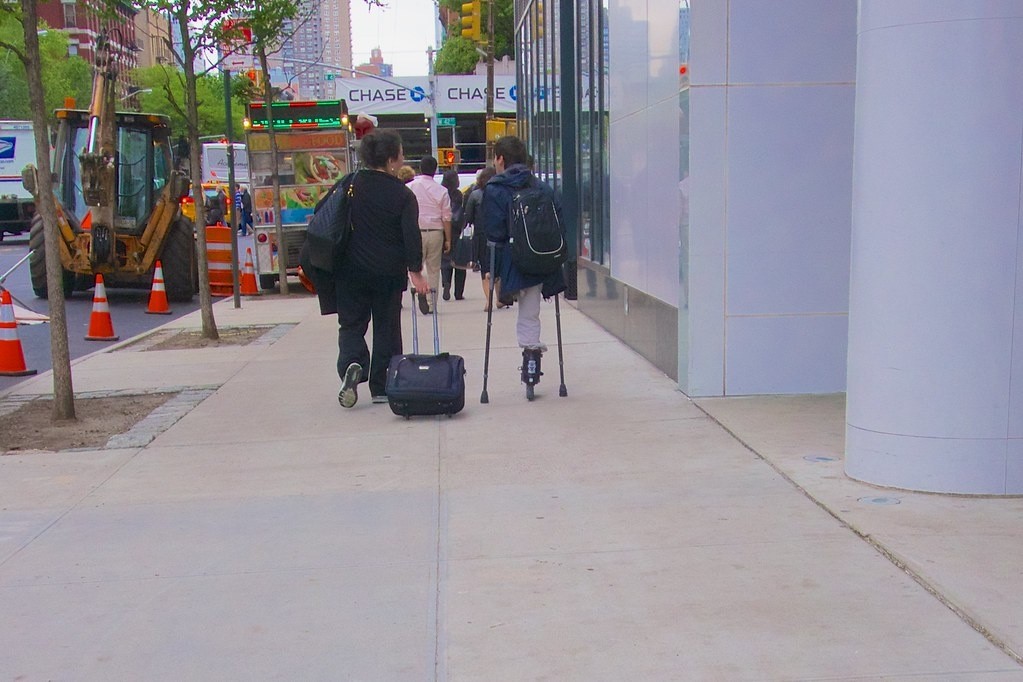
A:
[387,282,473,420]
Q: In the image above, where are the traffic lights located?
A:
[462,1,481,40]
[447,150,460,163]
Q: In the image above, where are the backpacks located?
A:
[498,179,571,273]
[303,170,363,287]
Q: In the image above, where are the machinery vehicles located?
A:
[22,27,198,304]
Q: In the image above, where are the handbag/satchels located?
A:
[451,222,479,270]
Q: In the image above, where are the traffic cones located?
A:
[240,248,263,297]
[0,290,38,376]
[145,260,172,314]
[84,274,119,341]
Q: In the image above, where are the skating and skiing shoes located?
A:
[521,351,541,399]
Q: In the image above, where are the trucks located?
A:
[0,120,57,244]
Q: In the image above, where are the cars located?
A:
[181,179,231,224]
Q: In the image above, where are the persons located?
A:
[207,185,229,227]
[583,150,619,300]
[397,156,452,314]
[314,129,431,408]
[234,184,253,236]
[482,136,568,386]
[440,153,535,311]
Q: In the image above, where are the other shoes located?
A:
[417,292,430,317]
[247,228,253,236]
[443,280,453,304]
[333,362,363,410]
